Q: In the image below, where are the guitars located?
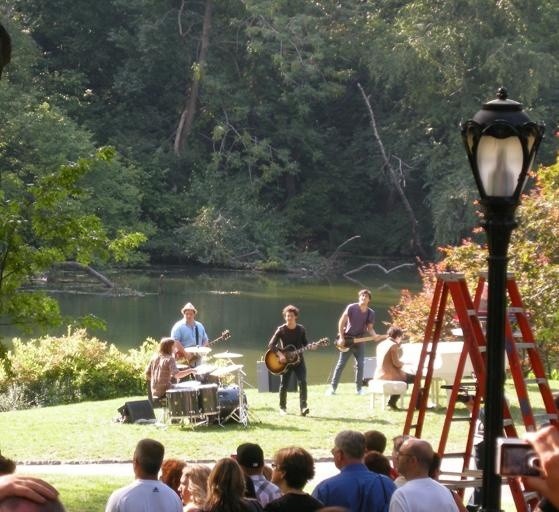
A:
[335,334,389,352]
[174,330,231,365]
[265,338,328,375]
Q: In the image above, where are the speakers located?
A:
[122,400,156,424]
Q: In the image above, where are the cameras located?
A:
[495,438,545,478]
[457,394,475,402]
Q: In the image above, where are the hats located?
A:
[181,302,198,315]
[230,444,263,468]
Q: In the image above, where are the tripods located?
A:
[220,369,263,428]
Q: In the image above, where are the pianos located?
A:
[398,342,472,408]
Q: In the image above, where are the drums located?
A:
[191,364,218,383]
[166,380,220,418]
[218,384,248,426]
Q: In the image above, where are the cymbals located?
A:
[174,364,188,369]
[184,347,213,353]
[209,364,244,376]
[213,352,243,358]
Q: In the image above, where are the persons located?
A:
[144,337,197,400]
[328,289,378,395]
[171,302,208,354]
[0,474,60,504]
[268,304,310,415]
[375,326,423,411]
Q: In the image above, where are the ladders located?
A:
[400,271,557,510]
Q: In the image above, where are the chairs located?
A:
[147,383,180,428]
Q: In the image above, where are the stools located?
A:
[367,379,408,414]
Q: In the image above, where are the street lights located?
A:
[456,84,550,512]
